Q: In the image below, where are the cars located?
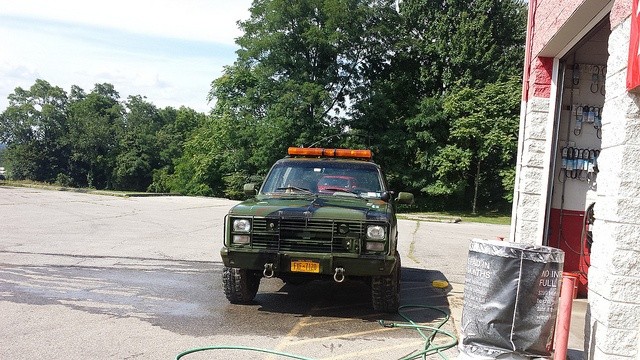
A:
[317,175,356,194]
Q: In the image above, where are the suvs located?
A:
[220,147,414,312]
